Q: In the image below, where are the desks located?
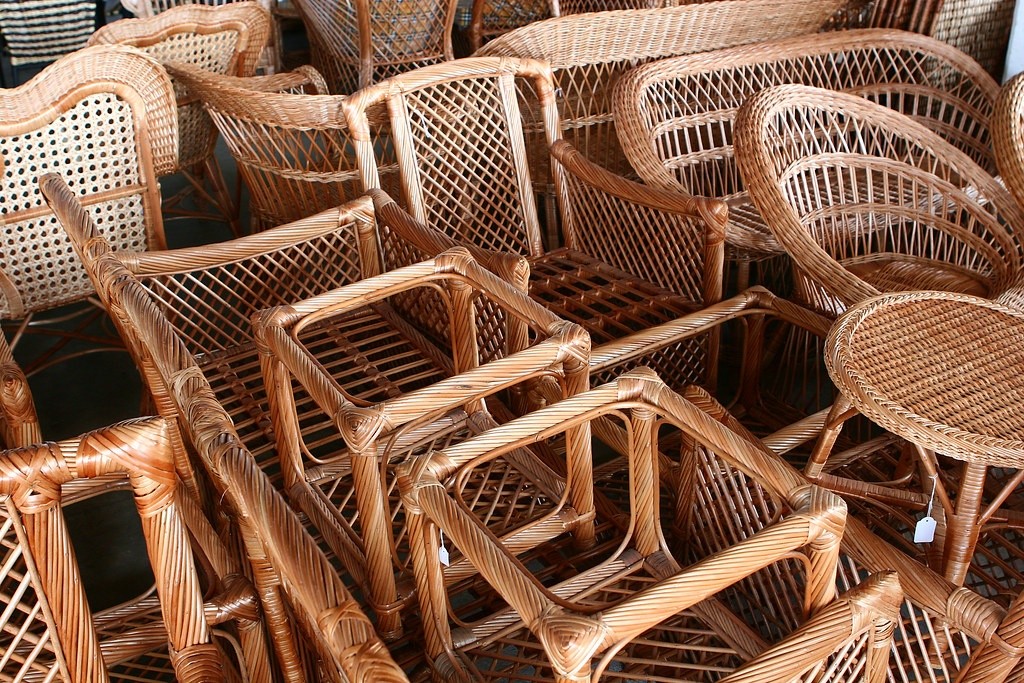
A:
[812,289,1024,673]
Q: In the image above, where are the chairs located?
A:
[164,58,408,234]
[733,78,1024,411]
[81,2,274,238]
[342,55,732,471]
[292,0,460,96]
[0,42,180,379]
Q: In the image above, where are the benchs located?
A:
[467,0,854,240]
[611,23,1006,388]
[37,170,904,683]
